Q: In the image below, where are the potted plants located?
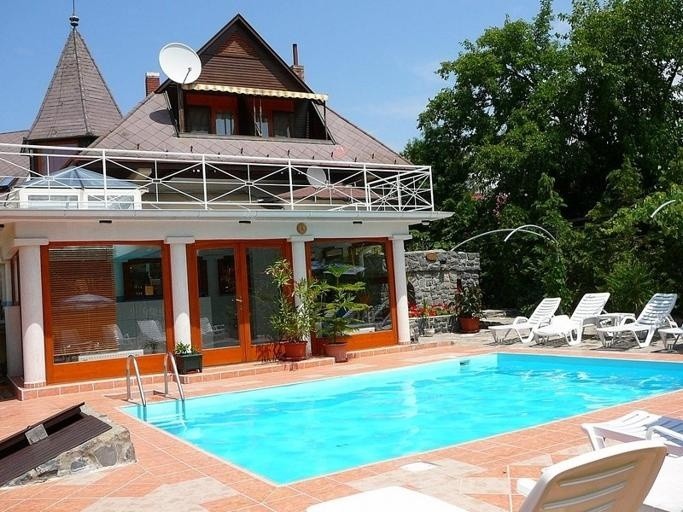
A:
[456,282,484,332]
[260,262,324,364]
[312,261,372,364]
[171,344,204,376]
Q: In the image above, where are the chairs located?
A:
[582,410,683,460]
[58,315,226,360]
[489,292,681,352]
[300,440,667,511]
